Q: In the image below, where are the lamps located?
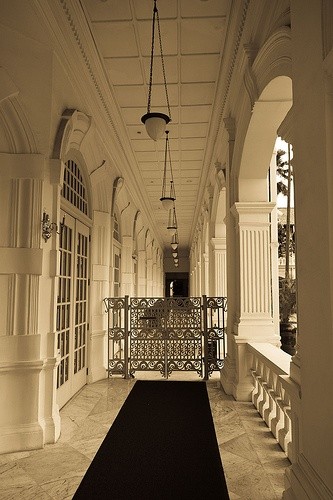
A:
[42,209,66,244]
[141,0,173,142]
[158,131,177,211]
[167,180,179,270]
